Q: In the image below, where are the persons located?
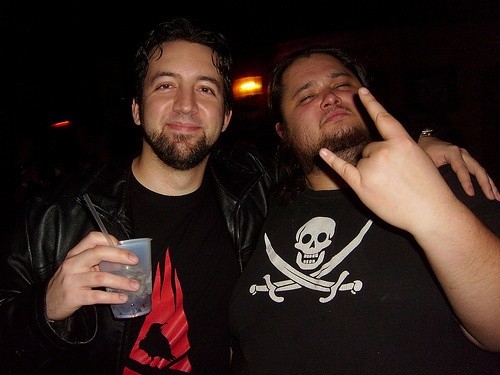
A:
[2,30,500,374]
[236,45,499,375]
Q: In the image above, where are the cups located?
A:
[98,237,153,319]
